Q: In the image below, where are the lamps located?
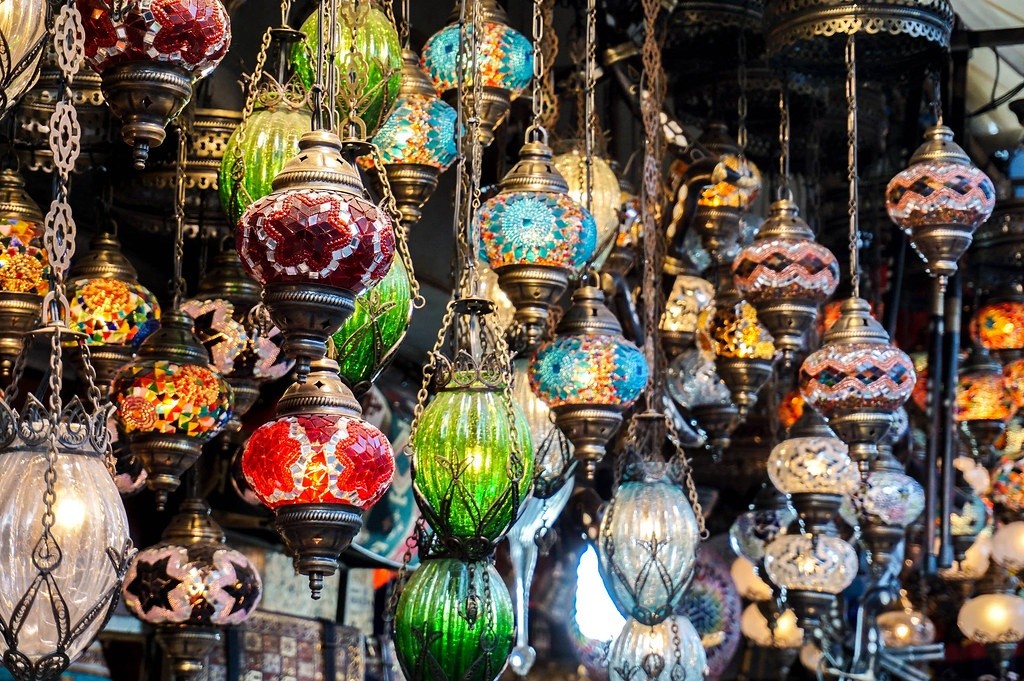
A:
[0,0,1024,681]
